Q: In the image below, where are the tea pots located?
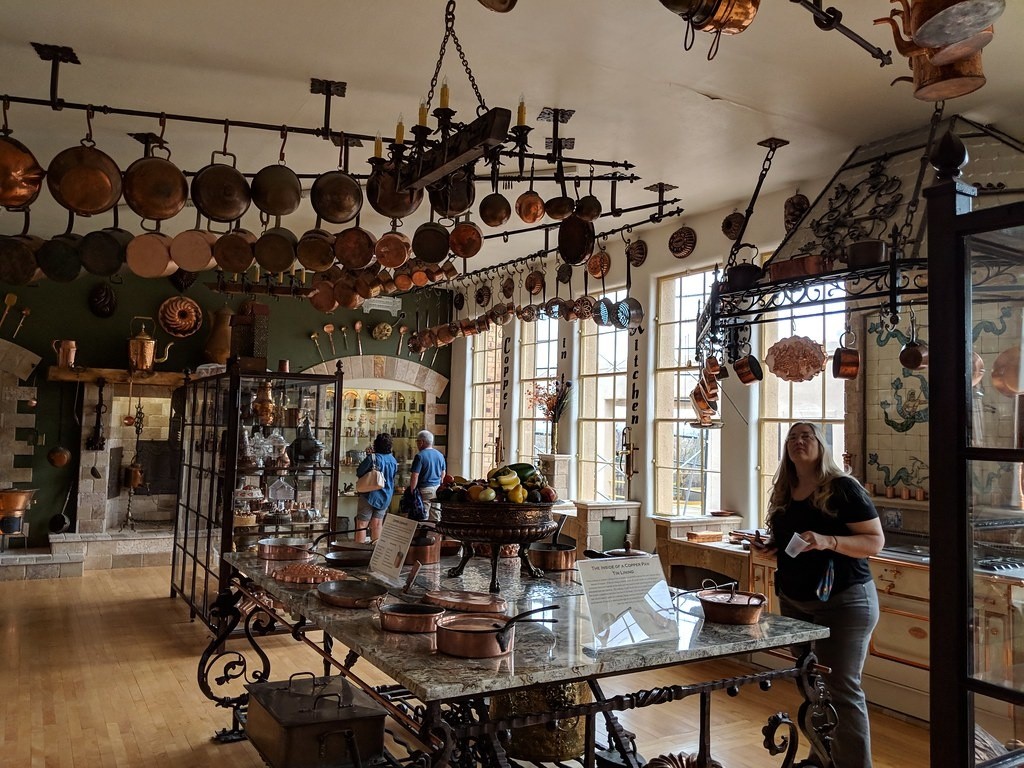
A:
[127,316,174,371]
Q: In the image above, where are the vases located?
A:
[551,423,558,454]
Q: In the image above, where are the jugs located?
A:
[52,338,77,367]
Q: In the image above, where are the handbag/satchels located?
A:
[357,452,386,492]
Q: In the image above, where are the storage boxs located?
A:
[244,675,388,768]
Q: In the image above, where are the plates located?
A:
[710,511,736,516]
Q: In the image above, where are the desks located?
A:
[198,552,839,768]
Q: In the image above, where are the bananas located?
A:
[488,466,521,490]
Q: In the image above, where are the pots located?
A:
[436,604,560,659]
[2,98,644,355]
[257,527,370,561]
[844,236,889,270]
[696,579,767,625]
[583,540,648,559]
[316,579,389,608]
[521,514,576,572]
[404,522,441,565]
[376,596,446,633]
[280,542,374,567]
[237,380,325,468]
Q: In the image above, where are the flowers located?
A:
[525,373,574,423]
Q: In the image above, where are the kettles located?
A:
[726,242,763,291]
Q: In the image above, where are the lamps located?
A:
[365,0,535,213]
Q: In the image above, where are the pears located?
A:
[507,482,528,504]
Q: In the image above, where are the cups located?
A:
[785,531,810,558]
[863,482,925,502]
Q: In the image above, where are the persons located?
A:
[354,433,397,543]
[764,421,885,767]
[399,430,446,521]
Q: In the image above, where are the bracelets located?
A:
[832,536,838,551]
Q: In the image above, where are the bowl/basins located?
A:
[991,346,1024,398]
[898,340,929,370]
[832,347,861,380]
[732,354,764,386]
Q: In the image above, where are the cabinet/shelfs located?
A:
[748,543,1024,749]
[170,353,344,654]
